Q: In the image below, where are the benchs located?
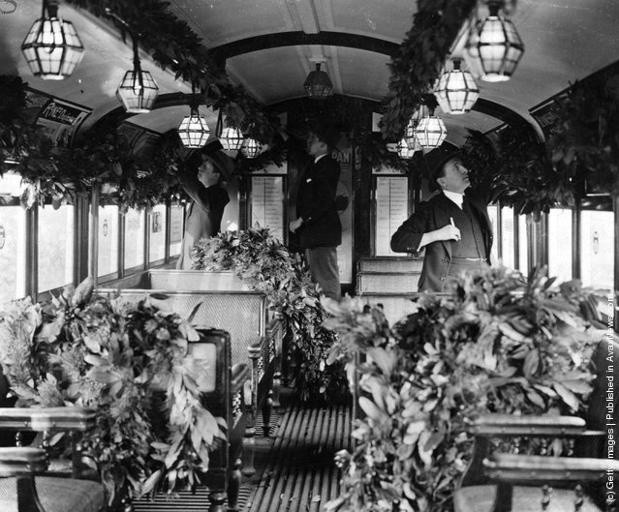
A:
[0,270,288,512]
[348,255,618,512]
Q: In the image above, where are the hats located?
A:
[200,150,238,177]
[418,141,460,179]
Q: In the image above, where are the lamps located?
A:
[242,128,262,161]
[219,118,244,152]
[22,1,84,81]
[178,78,211,150]
[303,55,333,101]
[117,36,158,114]
[386,0,524,159]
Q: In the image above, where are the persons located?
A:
[390,145,496,310]
[279,118,352,347]
[169,149,234,272]
